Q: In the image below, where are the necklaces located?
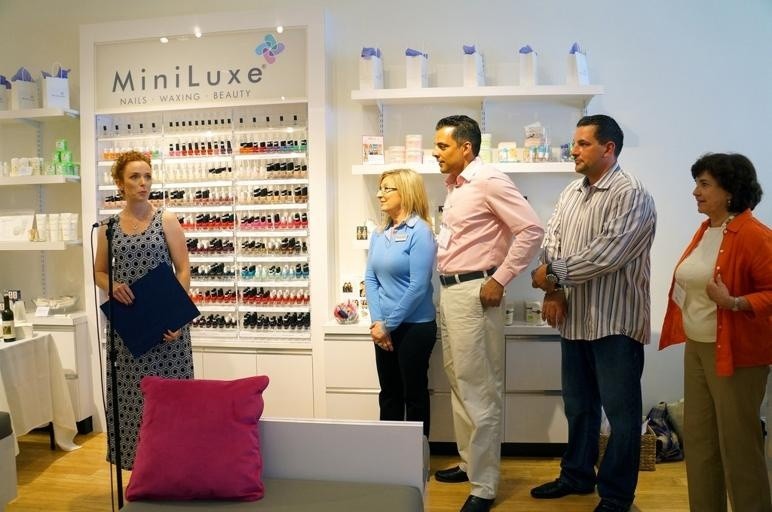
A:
[122,204,152,232]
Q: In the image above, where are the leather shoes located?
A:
[530,477,596,500]
[459,494,496,512]
[435,465,469,483]
[593,499,632,512]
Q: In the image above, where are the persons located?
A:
[362,169,438,440]
[431,115,545,512]
[530,113,657,512]
[658,152,768,512]
[93,150,196,470]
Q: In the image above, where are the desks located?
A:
[0,330,83,451]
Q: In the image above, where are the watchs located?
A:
[545,261,560,286]
[732,295,742,315]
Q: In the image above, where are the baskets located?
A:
[596,424,657,469]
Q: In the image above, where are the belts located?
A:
[439,268,496,286]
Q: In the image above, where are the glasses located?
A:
[378,186,397,193]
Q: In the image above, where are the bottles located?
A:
[2,293,16,343]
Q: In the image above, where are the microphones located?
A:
[92,213,120,228]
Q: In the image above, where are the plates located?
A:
[31,295,80,309]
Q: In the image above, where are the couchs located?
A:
[116,419,424,511]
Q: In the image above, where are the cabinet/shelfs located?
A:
[257,355,313,419]
[326,318,569,444]
[351,85,607,251]
[203,352,257,385]
[0,108,80,249]
[96,98,306,342]
[27,315,92,433]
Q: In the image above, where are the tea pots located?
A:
[333,292,361,324]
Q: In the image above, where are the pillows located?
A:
[125,374,270,501]
